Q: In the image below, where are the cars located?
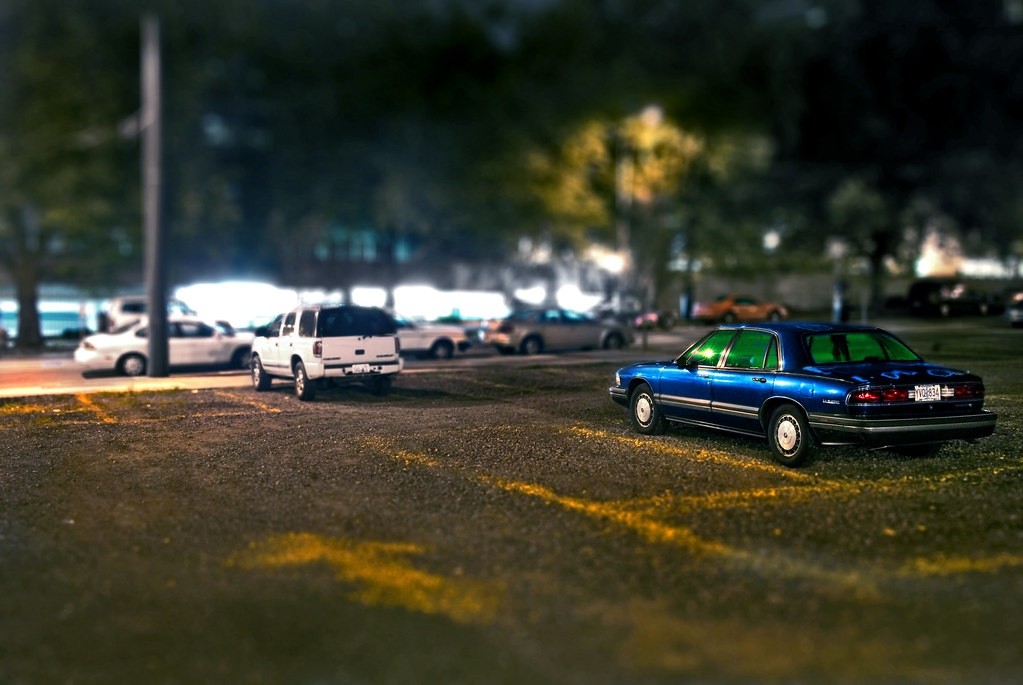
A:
[395,316,472,361]
[605,320,999,468]
[74,311,257,377]
[690,290,788,326]
[902,274,1023,328]
[475,302,638,355]
[97,293,198,332]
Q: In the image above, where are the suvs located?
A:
[246,301,407,401]
